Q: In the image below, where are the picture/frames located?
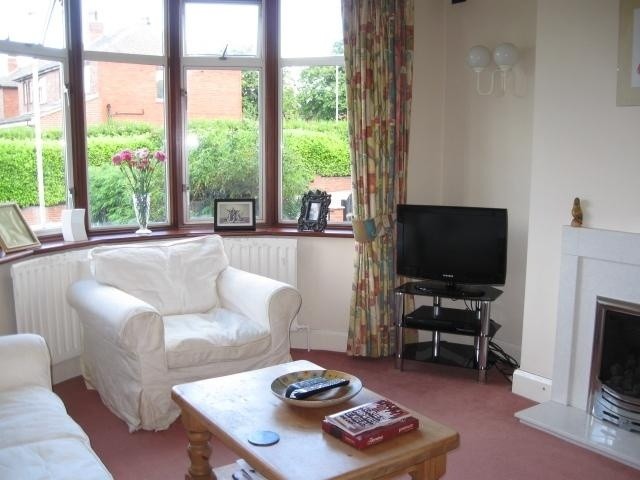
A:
[214,198,256,231]
[0,204,41,254]
[297,190,331,233]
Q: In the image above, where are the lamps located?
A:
[465,42,521,100]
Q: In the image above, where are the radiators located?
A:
[8,237,299,372]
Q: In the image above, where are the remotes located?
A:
[285,379,325,398]
[290,378,349,401]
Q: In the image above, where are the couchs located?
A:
[1,330,113,480]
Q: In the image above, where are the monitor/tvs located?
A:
[396,202,509,296]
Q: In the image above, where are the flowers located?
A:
[111,147,167,228]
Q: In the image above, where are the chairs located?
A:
[66,234,303,433]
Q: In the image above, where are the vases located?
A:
[132,193,153,234]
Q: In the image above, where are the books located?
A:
[322,396,419,450]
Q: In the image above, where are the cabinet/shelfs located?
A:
[392,281,504,384]
